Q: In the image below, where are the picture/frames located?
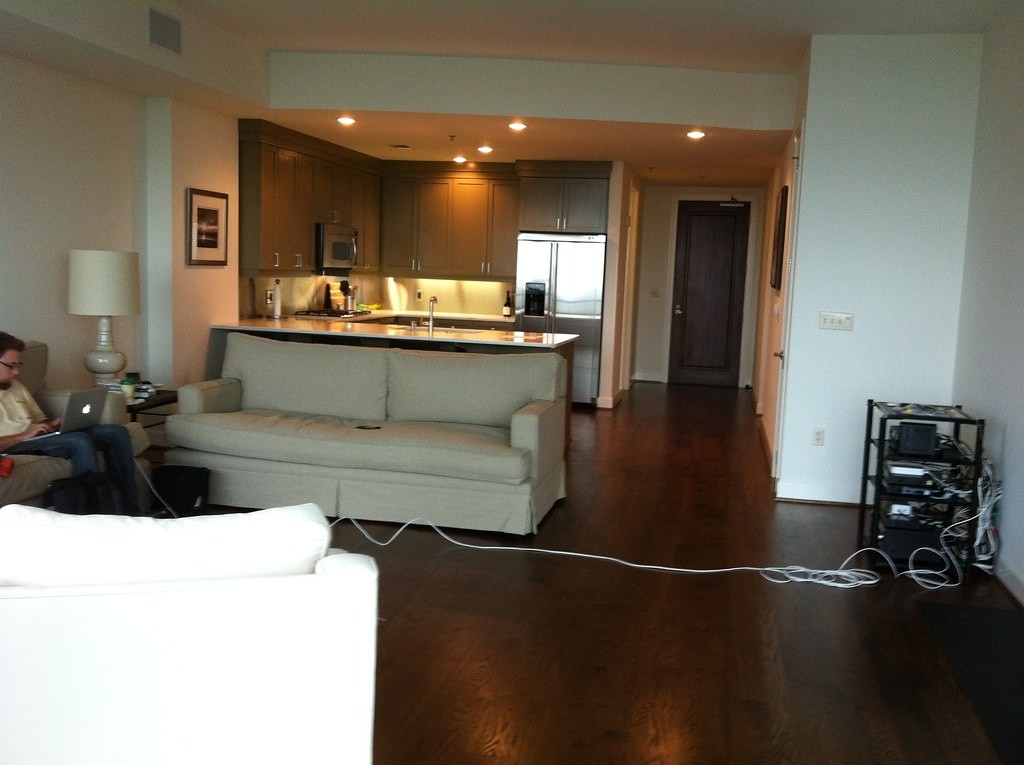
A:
[184,187,228,264]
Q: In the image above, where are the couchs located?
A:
[0,499,378,764]
[164,332,568,536]
[0,336,151,515]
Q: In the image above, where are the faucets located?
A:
[429,294,437,331]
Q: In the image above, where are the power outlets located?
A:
[820,313,853,330]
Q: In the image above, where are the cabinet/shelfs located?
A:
[859,398,987,573]
[236,116,610,286]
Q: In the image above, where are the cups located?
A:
[345,296,354,310]
[119,379,136,402]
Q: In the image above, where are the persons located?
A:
[0,332,136,490]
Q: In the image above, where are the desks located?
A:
[127,391,178,495]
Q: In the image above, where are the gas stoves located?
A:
[290,308,394,322]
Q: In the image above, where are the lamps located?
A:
[68,247,141,386]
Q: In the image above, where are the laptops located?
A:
[23,386,109,442]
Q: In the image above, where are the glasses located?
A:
[0,360,23,370]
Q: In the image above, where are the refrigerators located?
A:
[514,233,606,403]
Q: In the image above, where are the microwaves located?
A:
[314,223,359,270]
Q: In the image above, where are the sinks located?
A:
[402,326,487,335]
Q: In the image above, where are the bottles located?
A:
[503,290,511,316]
